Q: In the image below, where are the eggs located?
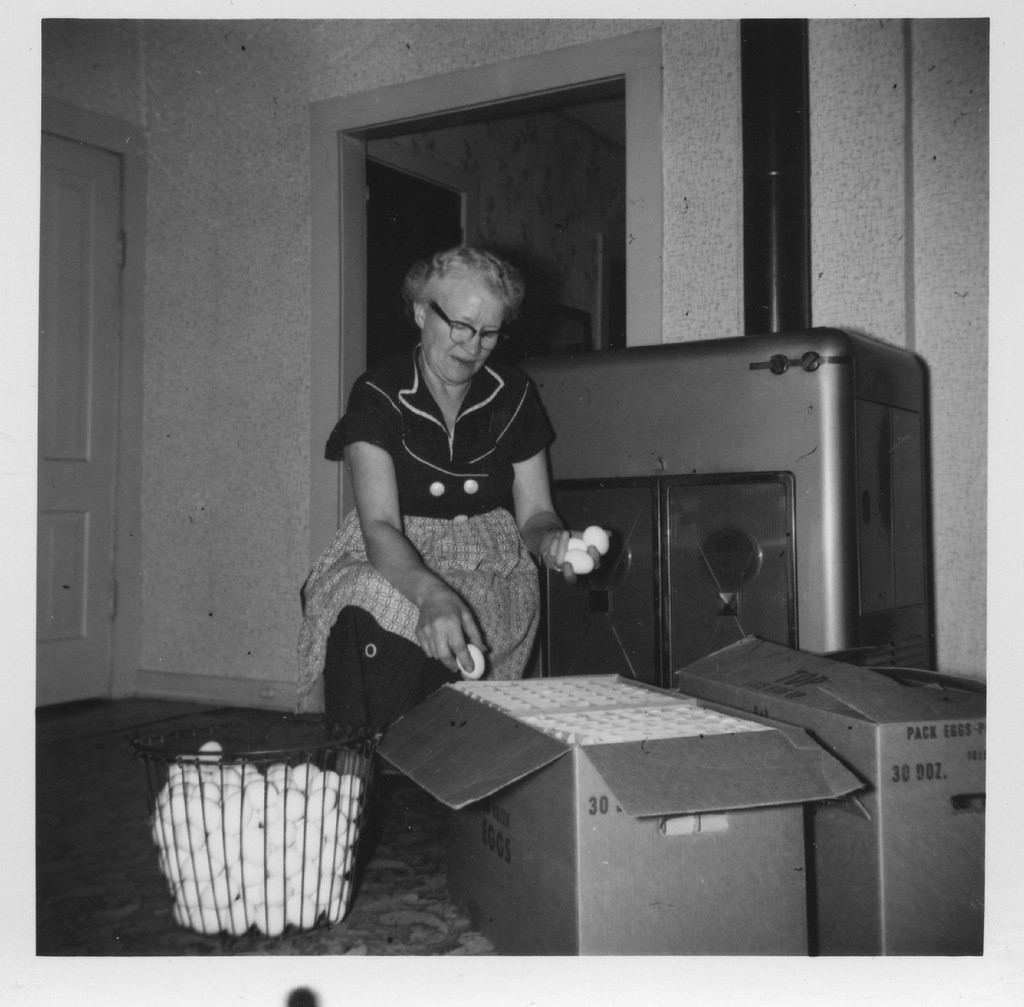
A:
[563,525,609,574]
[151,741,363,937]
[456,643,485,678]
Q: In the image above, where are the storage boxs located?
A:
[372,671,866,956]
[681,633,984,959]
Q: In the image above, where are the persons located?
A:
[299,241,613,850]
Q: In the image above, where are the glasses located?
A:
[430,301,512,352]
